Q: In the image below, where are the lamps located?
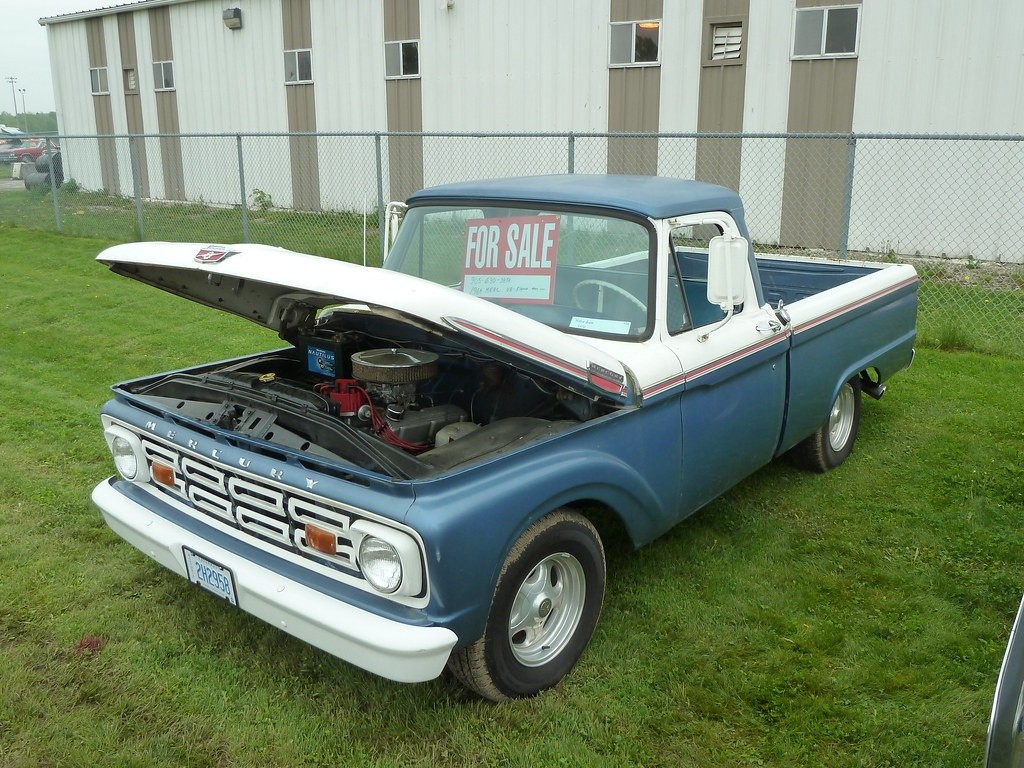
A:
[222,8,243,30]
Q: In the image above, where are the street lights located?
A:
[6,76,20,131]
[18,88,28,133]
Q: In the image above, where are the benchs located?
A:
[553,264,685,332]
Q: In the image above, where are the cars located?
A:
[0,138,61,166]
[89,171,922,709]
